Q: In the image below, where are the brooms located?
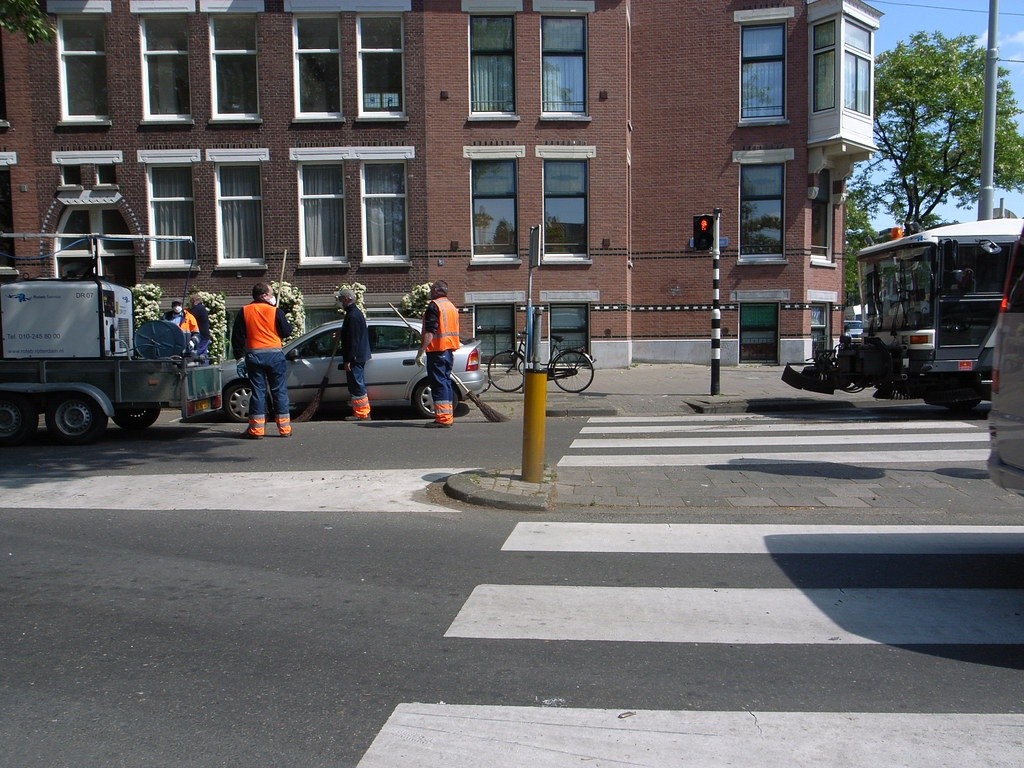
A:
[275,249,288,309]
[388,301,511,423]
[290,333,341,423]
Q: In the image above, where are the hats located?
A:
[335,289,355,300]
[172,299,182,303]
[429,280,448,293]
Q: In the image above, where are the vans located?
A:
[844,303,868,327]
[986,221,1024,502]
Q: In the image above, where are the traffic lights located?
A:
[692,215,712,255]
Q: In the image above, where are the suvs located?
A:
[844,320,863,344]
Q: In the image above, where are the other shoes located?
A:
[239,431,263,439]
[425,422,452,428]
[345,413,372,421]
[281,431,292,436]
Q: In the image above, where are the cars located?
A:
[223,317,491,422]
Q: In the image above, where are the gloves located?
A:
[200,355,205,361]
[236,359,247,378]
[416,349,426,368]
[188,339,197,351]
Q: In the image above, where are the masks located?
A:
[267,296,276,305]
[173,306,182,313]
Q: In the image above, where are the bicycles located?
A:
[488,325,597,392]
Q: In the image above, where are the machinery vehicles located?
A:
[780,218,1024,412]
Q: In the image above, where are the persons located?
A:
[231,281,292,439]
[162,298,200,361]
[416,280,459,428]
[339,289,372,420]
[186,293,210,366]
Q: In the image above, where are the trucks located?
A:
[0,266,223,449]
